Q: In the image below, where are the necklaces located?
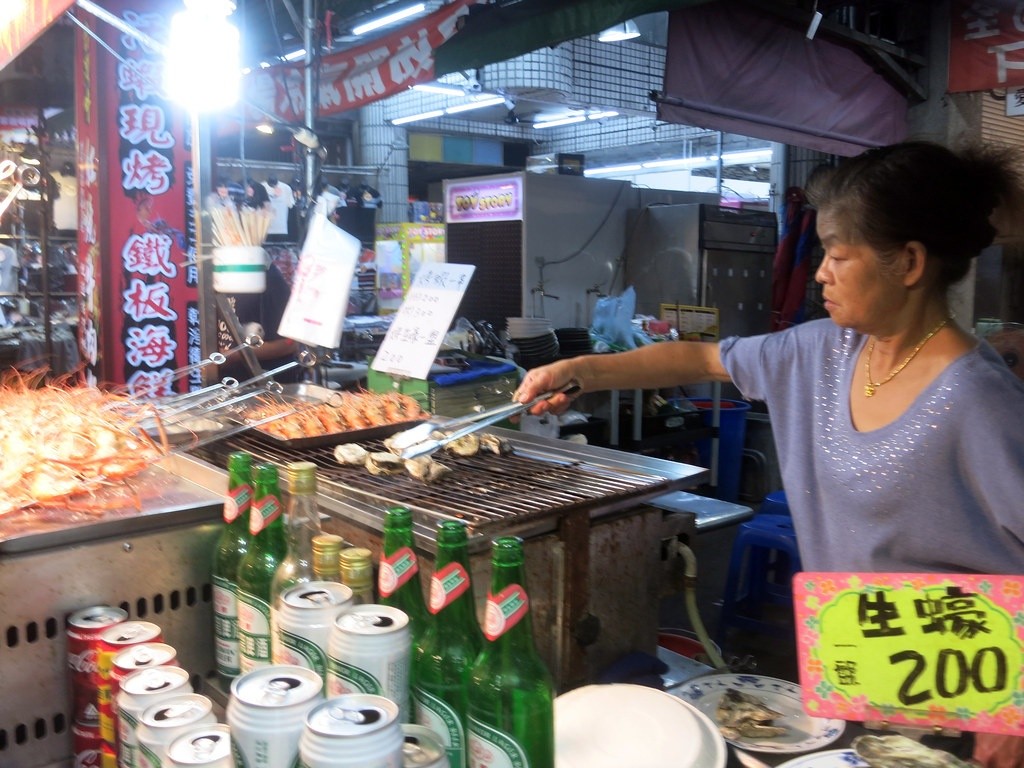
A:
[865,318,954,396]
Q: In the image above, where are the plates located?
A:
[553,682,729,768]
[774,749,887,768]
[664,673,845,755]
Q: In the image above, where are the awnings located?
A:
[433,0,681,89]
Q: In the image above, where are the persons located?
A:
[218,245,303,384]
[508,142,1023,768]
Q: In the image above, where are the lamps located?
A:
[390,109,444,126]
[445,96,506,114]
[530,109,620,129]
[597,18,642,41]
[352,4,426,34]
[413,83,467,99]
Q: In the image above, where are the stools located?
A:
[759,489,802,585]
[713,511,809,666]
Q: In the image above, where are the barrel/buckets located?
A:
[669,397,752,505]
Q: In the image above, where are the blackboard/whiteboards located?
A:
[370,261,476,381]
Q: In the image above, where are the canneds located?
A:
[117,666,194,768]
[324,603,412,723]
[297,693,405,768]
[97,620,165,768]
[65,605,129,727]
[225,665,325,767]
[110,642,180,767]
[273,579,353,698]
[69,723,117,768]
[134,693,218,768]
[164,723,235,767]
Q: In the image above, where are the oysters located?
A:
[333,443,370,466]
[715,686,792,738]
[364,452,410,477]
[434,428,518,457]
[849,733,981,768]
[403,453,455,483]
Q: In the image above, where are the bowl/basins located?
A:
[504,316,596,371]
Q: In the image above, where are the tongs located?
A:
[388,379,580,463]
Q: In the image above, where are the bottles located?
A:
[209,452,556,768]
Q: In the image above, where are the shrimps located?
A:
[245,386,432,438]
[0,363,174,516]
